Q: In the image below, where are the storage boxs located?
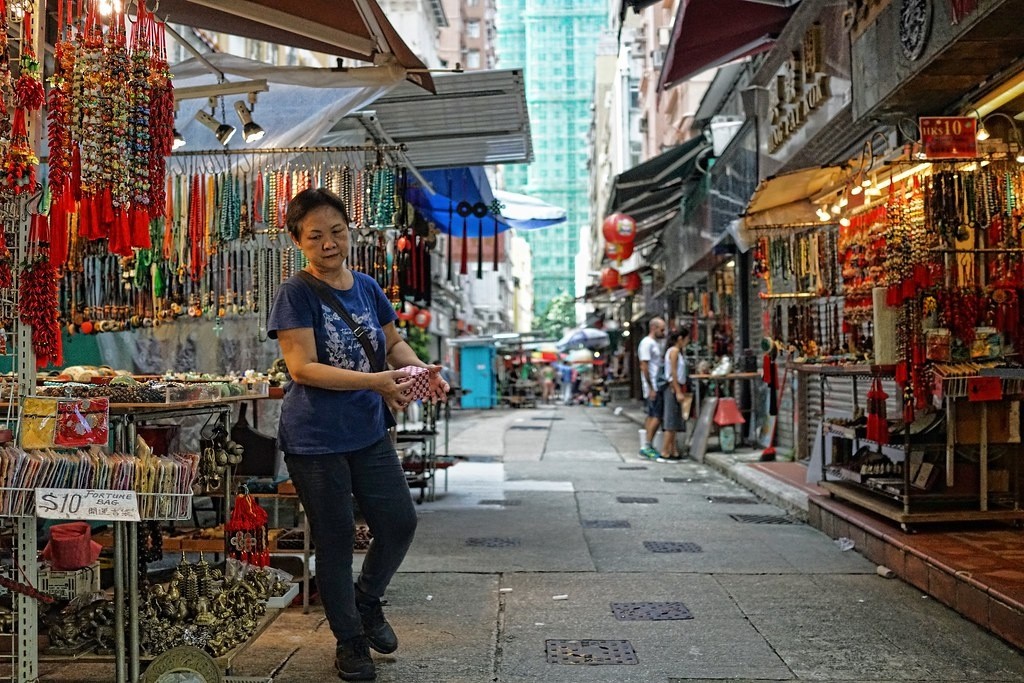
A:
[393,365,430,402]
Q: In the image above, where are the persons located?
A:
[638,317,689,464]
[267,187,451,682]
[432,359,454,421]
[503,351,628,406]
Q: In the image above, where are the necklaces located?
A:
[58,149,436,333]
[921,162,1024,240]
[752,225,837,297]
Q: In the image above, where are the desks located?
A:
[104,395,259,683]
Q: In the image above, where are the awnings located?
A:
[607,137,710,252]
[657,0,797,93]
[135,0,570,237]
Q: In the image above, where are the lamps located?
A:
[235,91,266,144]
[195,97,237,146]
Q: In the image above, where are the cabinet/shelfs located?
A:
[787,363,1024,533]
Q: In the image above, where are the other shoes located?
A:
[637,448,660,461]
[656,454,690,463]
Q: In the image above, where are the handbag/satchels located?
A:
[384,402,397,430]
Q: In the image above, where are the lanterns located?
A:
[600,269,639,292]
[603,212,634,260]
[396,301,430,330]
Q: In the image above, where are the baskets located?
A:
[0,486,194,520]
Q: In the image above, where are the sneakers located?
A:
[353,582,398,654]
[335,636,375,678]
